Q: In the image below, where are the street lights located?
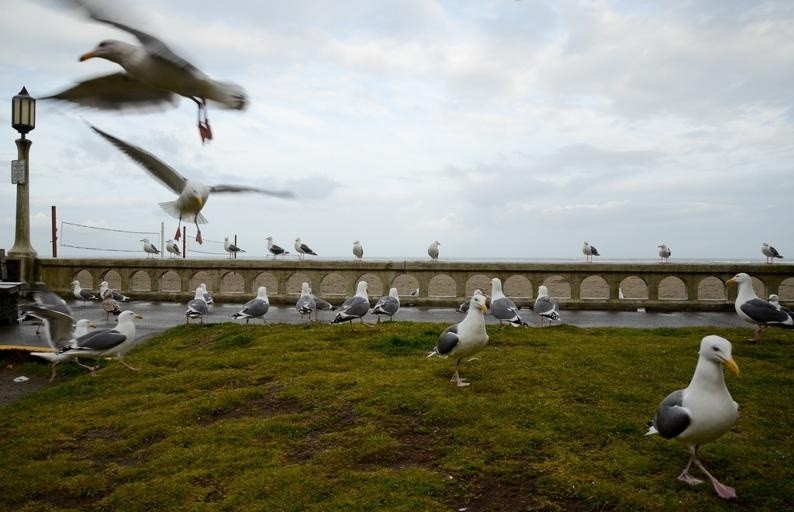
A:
[7,86,36,257]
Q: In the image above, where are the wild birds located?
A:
[645,335,740,498]
[725,242,794,343]
[428,240,440,261]
[184,236,399,325]
[456,278,562,330]
[410,288,419,296]
[15,280,142,384]
[425,290,490,387]
[140,239,182,258]
[583,241,600,263]
[33,14,291,245]
[658,245,671,264]
